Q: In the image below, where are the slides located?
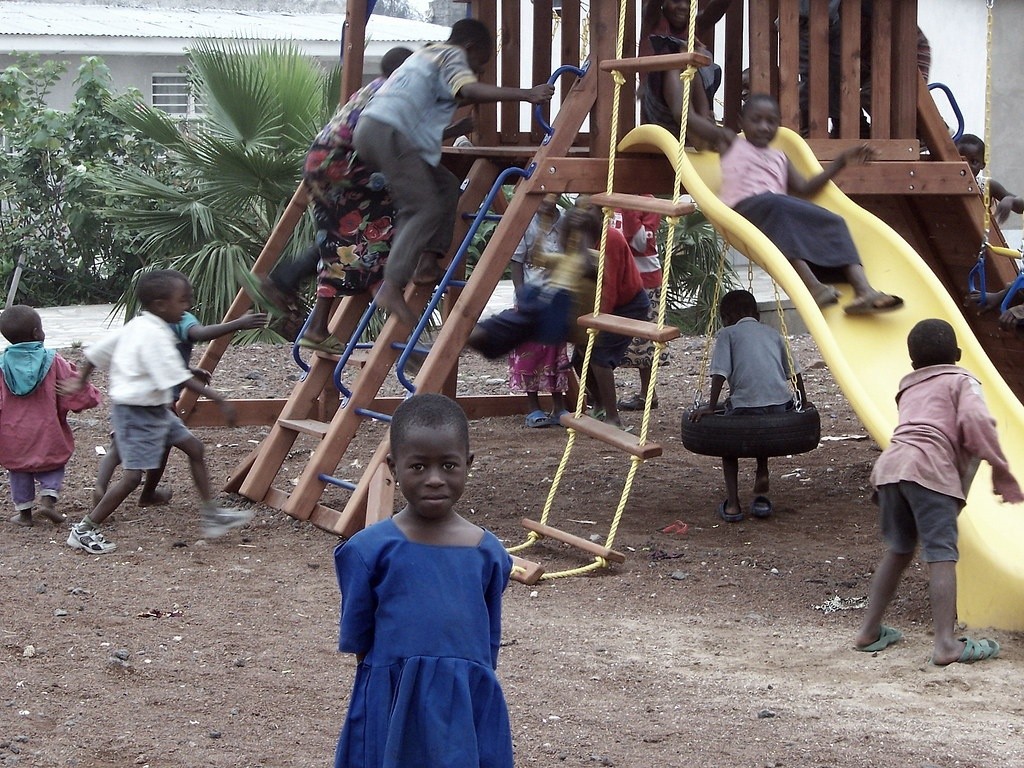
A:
[615,122,1024,633]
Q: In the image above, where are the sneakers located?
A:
[65,519,119,555]
[200,507,256,537]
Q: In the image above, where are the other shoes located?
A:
[843,291,904,314]
[620,396,659,411]
[817,285,840,308]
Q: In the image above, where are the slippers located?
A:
[297,330,353,354]
[526,410,568,428]
[719,496,745,521]
[590,408,605,422]
[929,637,998,666]
[855,625,902,652]
[750,495,773,517]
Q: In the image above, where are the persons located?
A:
[954,134,1024,224]
[55,269,269,553]
[264,19,555,353]
[688,288,807,523]
[0,305,101,527]
[639,0,954,151]
[334,394,513,768]
[853,318,1024,666]
[466,194,661,429]
[664,45,903,316]
